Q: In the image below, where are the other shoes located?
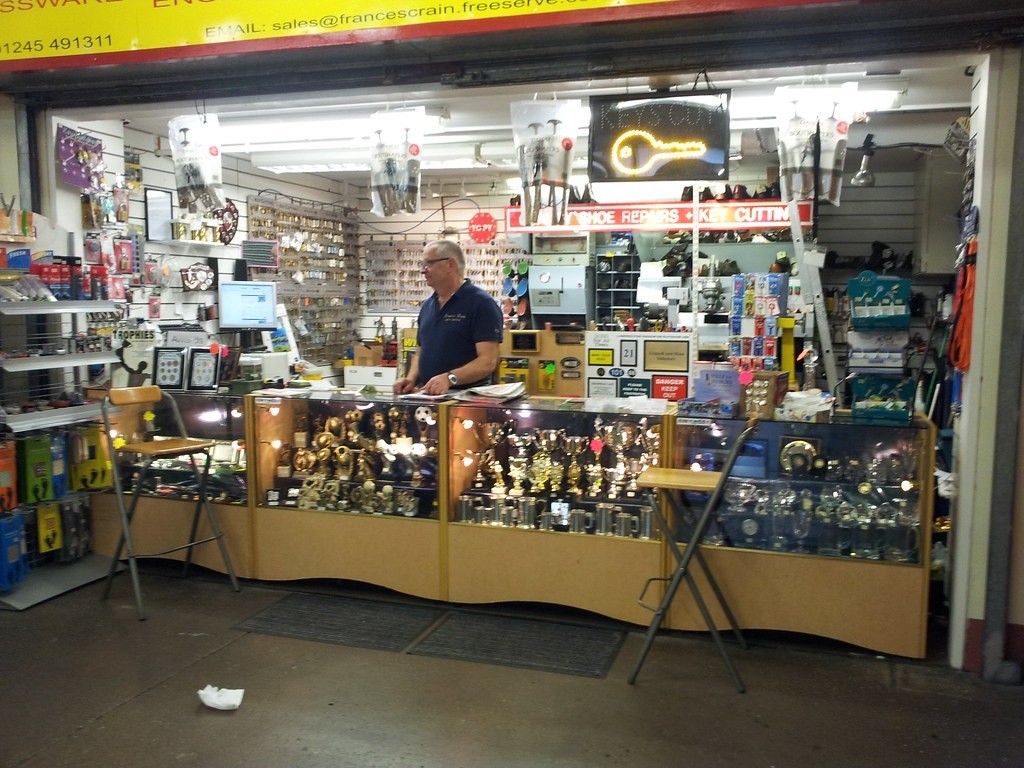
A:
[752,184,769,198]
[768,182,780,197]
[699,187,713,200]
[715,184,732,200]
[733,185,752,199]
[682,186,693,200]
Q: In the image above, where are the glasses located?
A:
[418,257,452,268]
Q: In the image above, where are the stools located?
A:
[629,425,758,692]
[101,385,243,622]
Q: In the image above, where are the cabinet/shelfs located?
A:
[0,300,129,612]
[914,149,964,277]
[342,366,399,393]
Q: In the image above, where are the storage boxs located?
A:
[353,345,385,366]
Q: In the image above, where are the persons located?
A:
[393,240,503,394]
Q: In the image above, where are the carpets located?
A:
[233,592,446,652]
[407,610,629,679]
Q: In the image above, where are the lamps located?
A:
[851,138,876,187]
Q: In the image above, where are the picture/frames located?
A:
[184,347,222,392]
[145,187,174,242]
[151,346,186,388]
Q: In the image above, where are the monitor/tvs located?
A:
[217,282,278,354]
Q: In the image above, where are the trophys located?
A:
[677,436,921,564]
[266,406,439,516]
[455,415,661,540]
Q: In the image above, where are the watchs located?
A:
[447,371,457,387]
